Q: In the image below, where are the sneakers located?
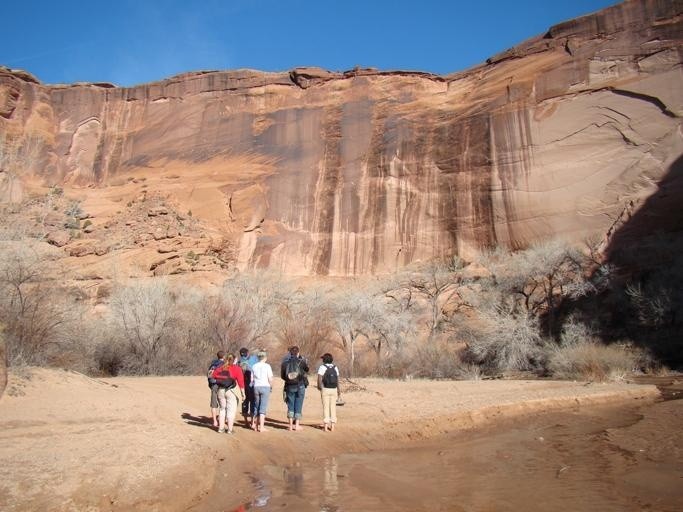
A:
[218,428,236,434]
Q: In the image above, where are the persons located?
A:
[207,350,225,427]
[319,453,341,511]
[235,347,256,430]
[248,350,273,432]
[280,345,309,431]
[211,352,246,433]
[316,353,341,431]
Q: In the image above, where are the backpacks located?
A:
[284,357,303,385]
[322,363,338,388]
